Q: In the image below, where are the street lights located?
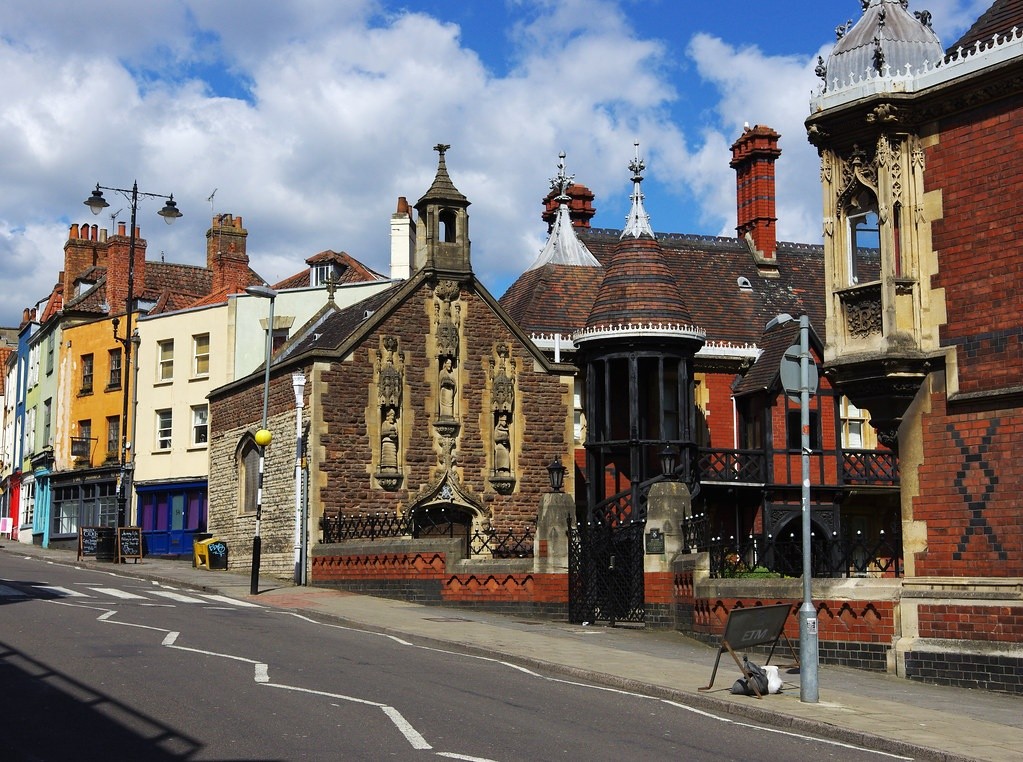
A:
[83,179,184,564]
[763,313,820,704]
[245,285,277,595]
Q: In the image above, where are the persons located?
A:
[494,414,510,476]
[381,408,399,472]
[439,359,456,417]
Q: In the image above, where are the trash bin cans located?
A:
[194,539,227,571]
[197,533,213,541]
[97,528,115,560]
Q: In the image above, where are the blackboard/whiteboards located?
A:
[118,527,142,557]
[80,526,100,555]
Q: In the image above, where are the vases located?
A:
[108,382,120,388]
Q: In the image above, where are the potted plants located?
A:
[106,450,118,461]
[80,384,91,394]
[74,456,89,466]
